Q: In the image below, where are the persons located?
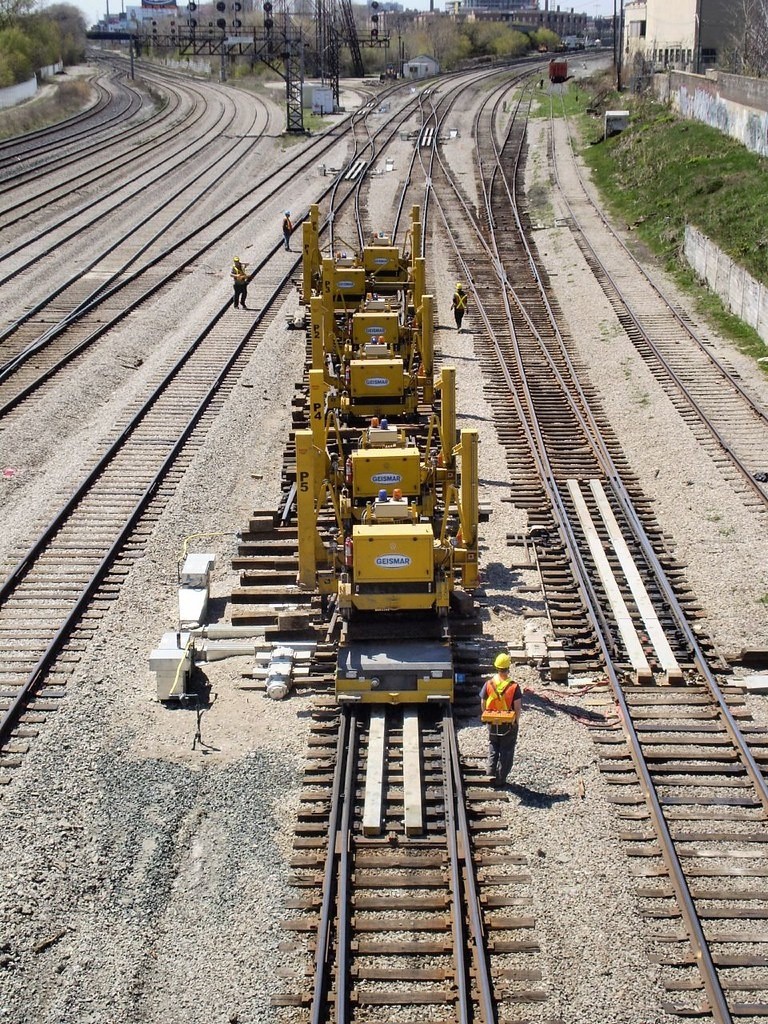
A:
[451,283,469,333]
[282,210,293,252]
[230,257,248,309]
[479,653,524,786]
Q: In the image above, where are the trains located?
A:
[549,58,567,83]
[295,205,479,706]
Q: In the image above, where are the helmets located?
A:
[456,283,462,289]
[285,211,291,215]
[234,257,239,261]
[494,653,511,668]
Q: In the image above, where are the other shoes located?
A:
[235,306,239,309]
[240,302,246,308]
[496,783,505,787]
[286,248,292,252]
[458,328,462,332]
[486,770,494,776]
